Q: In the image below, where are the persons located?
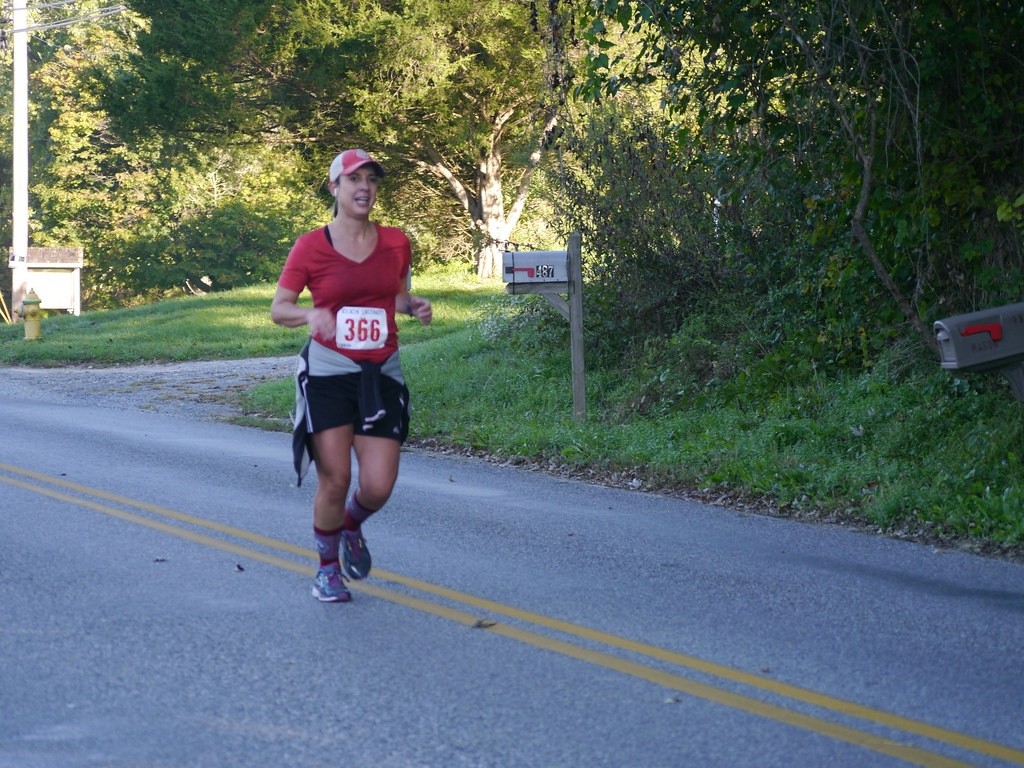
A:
[269,148,433,603]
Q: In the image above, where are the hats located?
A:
[329,149,383,182]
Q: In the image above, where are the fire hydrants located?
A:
[14,287,42,342]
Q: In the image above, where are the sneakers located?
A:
[311,568,351,602]
[341,530,371,579]
